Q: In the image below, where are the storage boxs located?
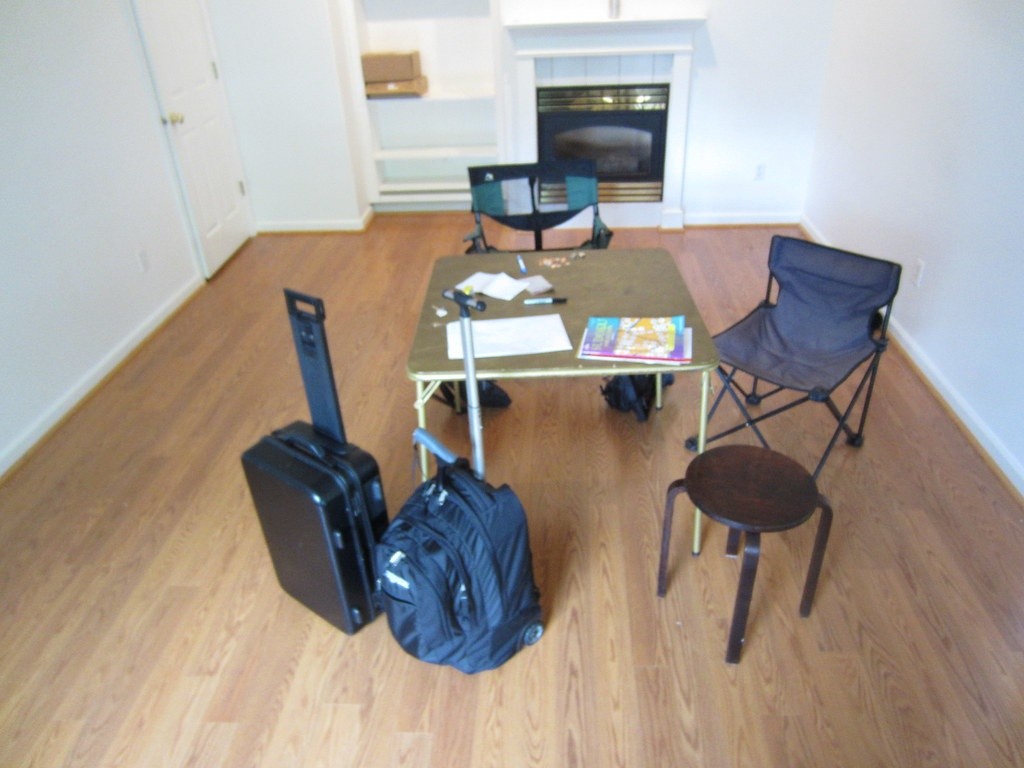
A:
[364,51,427,97]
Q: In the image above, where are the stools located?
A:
[657,444,834,664]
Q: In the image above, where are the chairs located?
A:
[685,236,903,484]
[464,155,613,256]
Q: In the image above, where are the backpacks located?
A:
[370,453,543,675]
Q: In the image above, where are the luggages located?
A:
[241,289,393,637]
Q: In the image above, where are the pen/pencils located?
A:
[516,255,527,273]
[524,298,568,305]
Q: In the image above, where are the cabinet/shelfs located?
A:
[498,0,708,31]
[367,94,502,205]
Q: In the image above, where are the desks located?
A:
[406,246,722,558]
